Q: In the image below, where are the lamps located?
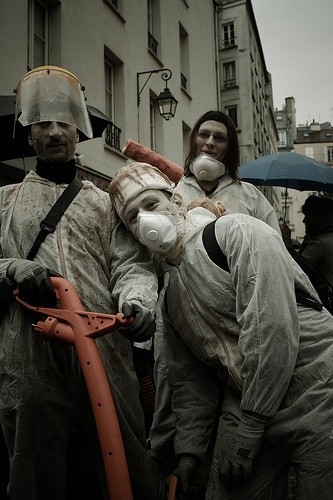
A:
[136,69,178,120]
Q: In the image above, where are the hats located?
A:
[107,163,175,229]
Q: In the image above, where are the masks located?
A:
[188,153,227,181]
[132,211,178,254]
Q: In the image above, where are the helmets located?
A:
[8,65,93,139]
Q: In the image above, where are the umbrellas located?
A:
[236,152,333,224]
[0,94,114,175]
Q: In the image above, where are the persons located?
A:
[0,65,333,500]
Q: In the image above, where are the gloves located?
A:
[168,456,194,492]
[122,300,157,344]
[7,259,64,306]
[222,413,264,479]
